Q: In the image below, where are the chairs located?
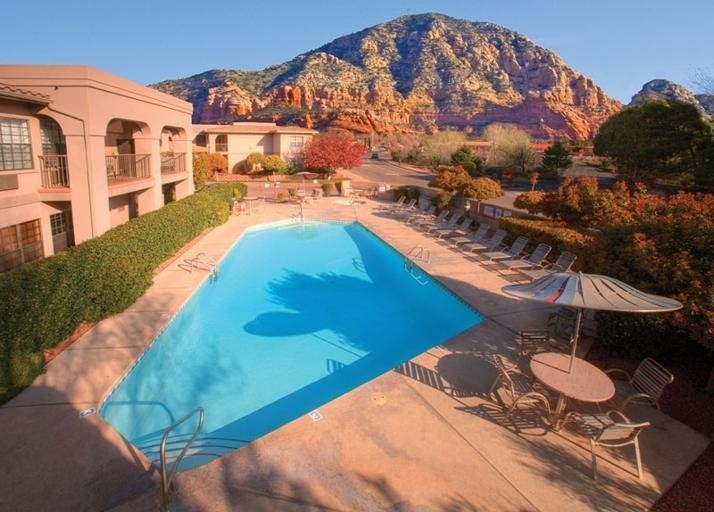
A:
[483,328,675,487]
[372,193,579,288]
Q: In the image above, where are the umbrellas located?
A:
[501,270,685,375]
[289,170,318,189]
